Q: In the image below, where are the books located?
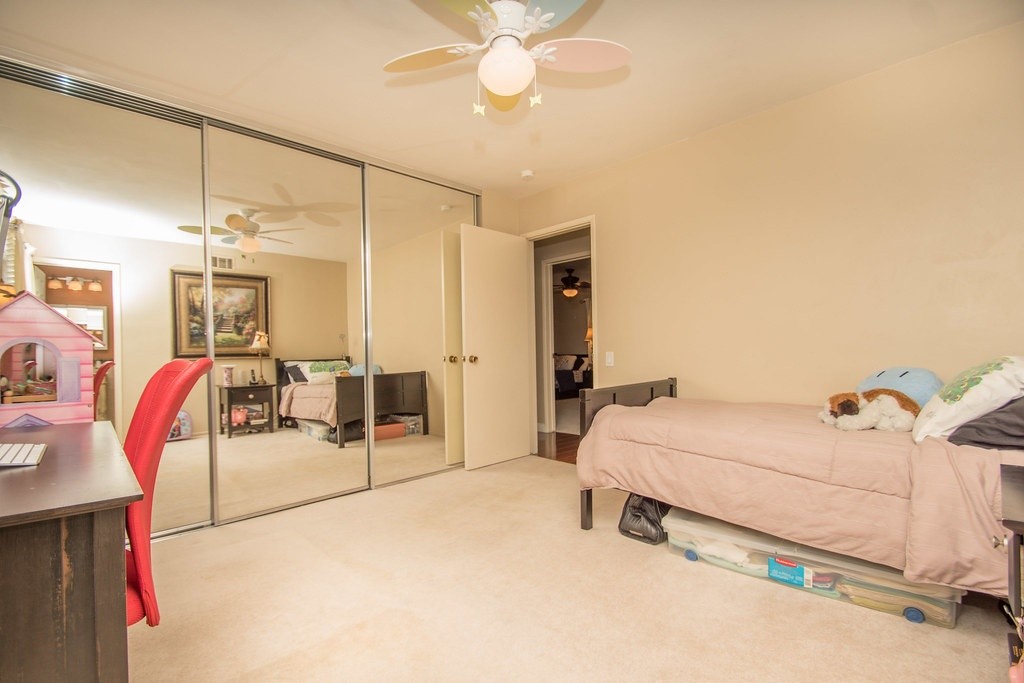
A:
[245,418,268,424]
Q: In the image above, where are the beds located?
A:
[577,377,1024,600]
[274,355,429,448]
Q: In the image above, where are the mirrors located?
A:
[362,162,478,491]
[200,116,370,528]
[0,55,213,547]
[48,303,109,351]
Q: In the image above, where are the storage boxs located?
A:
[294,418,331,442]
[375,419,406,441]
[389,414,423,438]
[660,505,969,629]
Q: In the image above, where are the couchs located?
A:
[555,352,593,391]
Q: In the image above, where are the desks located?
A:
[3,394,58,404]
[0,421,144,683]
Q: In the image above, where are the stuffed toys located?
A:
[857,367,945,410]
[818,387,921,432]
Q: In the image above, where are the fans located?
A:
[553,268,591,290]
[176,208,304,245]
[381,0,634,73]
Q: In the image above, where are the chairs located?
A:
[123,357,213,628]
[93,360,116,421]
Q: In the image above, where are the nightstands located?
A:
[215,384,276,439]
[999,464,1024,666]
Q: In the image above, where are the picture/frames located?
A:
[169,268,274,361]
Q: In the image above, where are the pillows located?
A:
[282,359,352,385]
[578,357,590,371]
[947,397,1024,452]
[555,356,577,370]
[296,360,351,386]
[284,366,309,382]
[911,354,1024,445]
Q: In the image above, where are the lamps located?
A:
[46,276,103,292]
[248,330,272,384]
[234,232,260,254]
[562,285,578,298]
[472,27,543,116]
